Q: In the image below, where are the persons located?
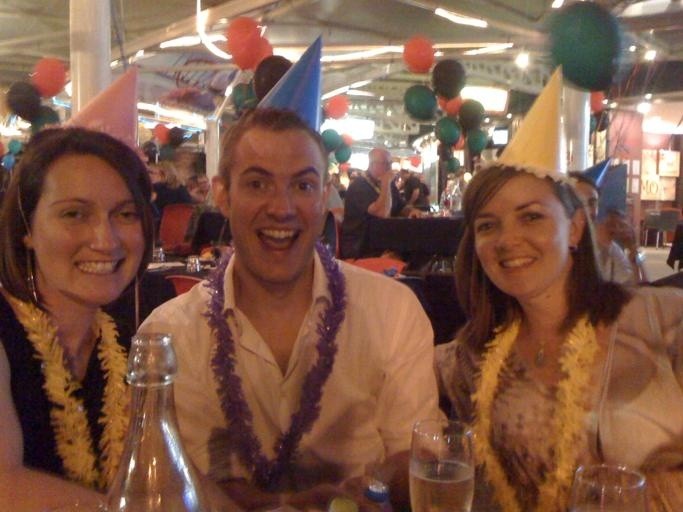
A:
[138,106,449,512]
[144,150,206,214]
[0,127,172,512]
[323,148,430,218]
[437,162,683,512]
[566,171,640,284]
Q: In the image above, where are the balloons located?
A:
[587,115,597,134]
[318,128,339,154]
[6,79,39,123]
[595,110,610,134]
[337,133,353,142]
[324,94,348,119]
[543,6,628,97]
[30,56,67,102]
[222,14,291,111]
[7,140,21,154]
[590,92,605,114]
[138,124,187,163]
[29,107,58,128]
[402,37,487,157]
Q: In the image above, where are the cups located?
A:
[186,255,202,273]
[564,465,649,512]
[407,417,477,512]
[156,248,168,272]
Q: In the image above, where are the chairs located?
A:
[640,207,683,250]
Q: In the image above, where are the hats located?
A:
[496,63,568,175]
[256,34,321,136]
[582,158,610,188]
[64,66,139,147]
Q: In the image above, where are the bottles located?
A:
[439,180,462,214]
[104,336,209,512]
